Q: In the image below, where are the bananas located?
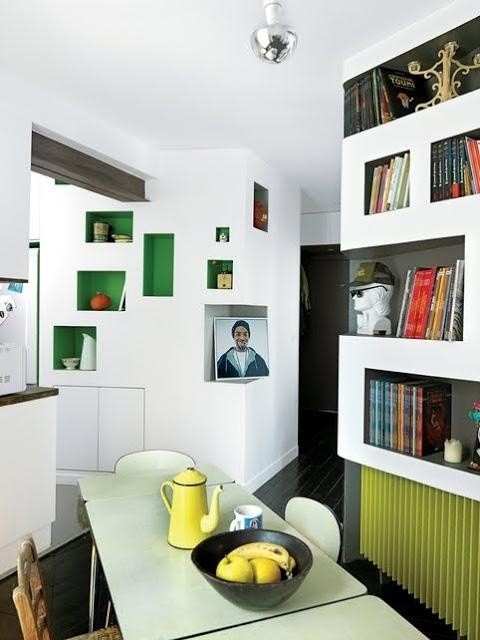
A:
[228,541,297,577]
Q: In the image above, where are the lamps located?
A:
[250,0,298,65]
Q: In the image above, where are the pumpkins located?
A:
[90,292,111,309]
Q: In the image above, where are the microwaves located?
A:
[0,343,30,396]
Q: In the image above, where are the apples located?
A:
[249,559,281,584]
[216,554,254,585]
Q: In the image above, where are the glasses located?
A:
[350,285,388,298]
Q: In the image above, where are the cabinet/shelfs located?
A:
[37,152,300,495]
[337,0,479,504]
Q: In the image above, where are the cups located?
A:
[92,220,110,242]
[442,440,462,464]
[228,504,265,532]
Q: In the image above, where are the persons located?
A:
[340,261,396,336]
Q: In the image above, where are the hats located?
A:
[231,320,251,339]
[340,261,396,287]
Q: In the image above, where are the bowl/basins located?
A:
[191,527,314,610]
[60,356,80,370]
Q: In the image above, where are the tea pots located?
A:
[157,468,224,551]
[80,331,96,370]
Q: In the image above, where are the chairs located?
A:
[12,537,123,640]
[284,496,343,567]
[104,449,195,629]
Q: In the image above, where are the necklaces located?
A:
[235,350,248,371]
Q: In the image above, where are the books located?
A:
[428,135,480,205]
[342,65,431,138]
[367,372,454,460]
[366,152,412,216]
[394,258,465,343]
